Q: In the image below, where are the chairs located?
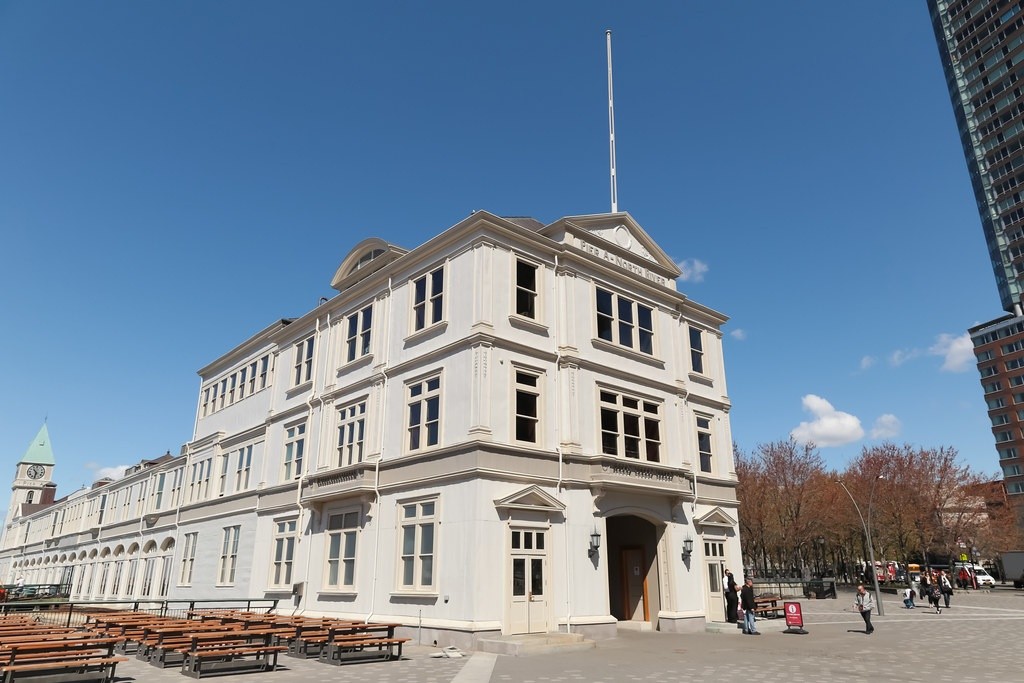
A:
[0,607,413,683]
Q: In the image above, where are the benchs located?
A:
[753,596,785,619]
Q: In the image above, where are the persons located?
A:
[855,585,875,635]
[928,579,943,614]
[740,578,760,635]
[900,567,977,610]
[723,567,730,605]
[727,572,741,623]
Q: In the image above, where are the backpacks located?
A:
[931,585,941,600]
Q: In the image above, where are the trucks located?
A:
[849,557,994,588]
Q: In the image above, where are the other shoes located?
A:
[870,627,874,633]
[905,602,954,616]
[865,629,870,634]
[727,613,763,636]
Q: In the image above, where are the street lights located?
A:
[965,538,978,591]
[833,474,886,617]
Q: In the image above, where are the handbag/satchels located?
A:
[949,588,953,595]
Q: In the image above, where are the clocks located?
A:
[26,464,46,480]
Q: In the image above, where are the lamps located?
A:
[681,539,693,560]
[588,532,601,558]
